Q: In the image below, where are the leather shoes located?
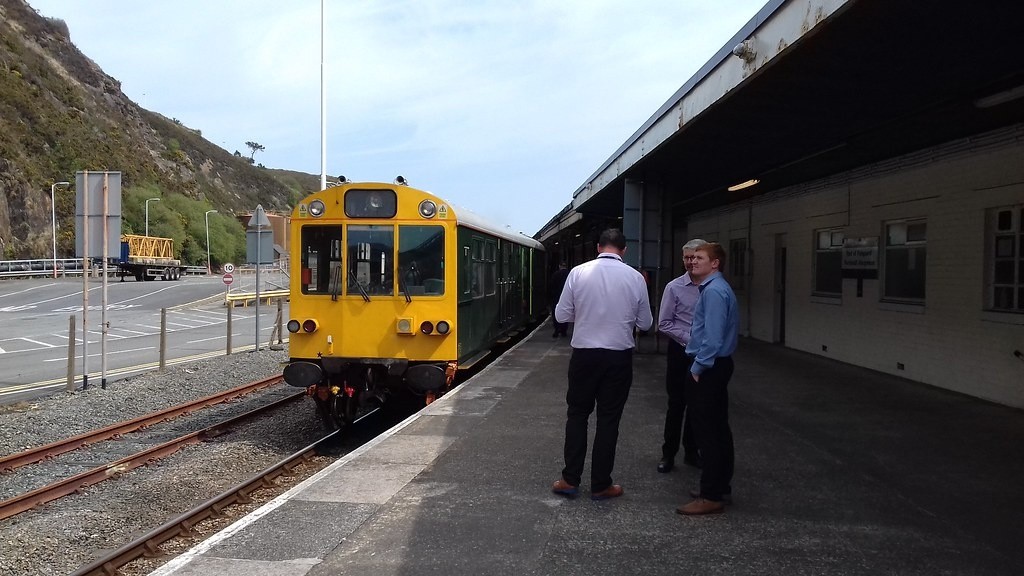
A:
[552,479,579,494]
[683,453,704,469]
[657,456,675,472]
[689,488,701,498]
[591,484,623,500]
[676,497,724,515]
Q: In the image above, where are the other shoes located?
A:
[561,332,567,337]
[553,329,560,338]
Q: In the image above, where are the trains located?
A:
[283,174,552,433]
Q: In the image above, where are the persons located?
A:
[553,227,654,500]
[551,261,569,339]
[675,241,741,518]
[656,239,704,474]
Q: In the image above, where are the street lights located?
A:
[51,182,70,278]
[146,198,161,237]
[206,209,220,275]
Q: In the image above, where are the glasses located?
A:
[682,255,695,262]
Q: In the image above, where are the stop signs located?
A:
[223,273,233,285]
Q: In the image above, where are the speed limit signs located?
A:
[224,263,235,273]
